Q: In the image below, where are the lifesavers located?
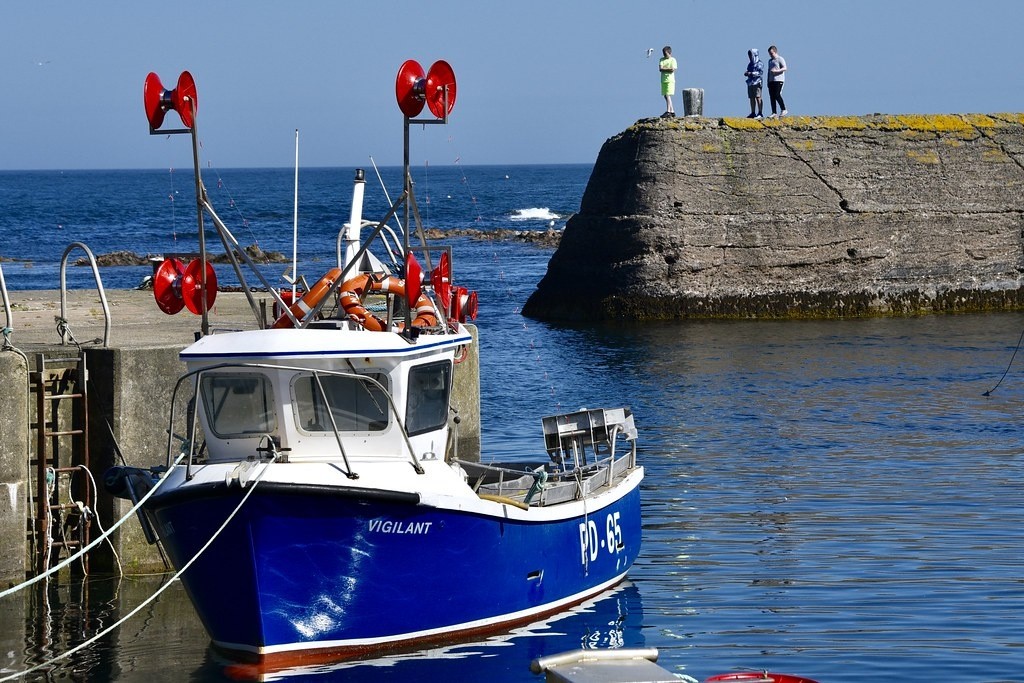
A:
[339,272,437,334]
[271,268,342,329]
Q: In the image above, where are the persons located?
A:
[744,49,764,119]
[766,46,788,118]
[659,46,677,118]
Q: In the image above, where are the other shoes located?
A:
[746,114,756,118]
[754,114,764,118]
[670,113,675,117]
[767,113,778,119]
[660,112,671,118]
[779,108,788,116]
